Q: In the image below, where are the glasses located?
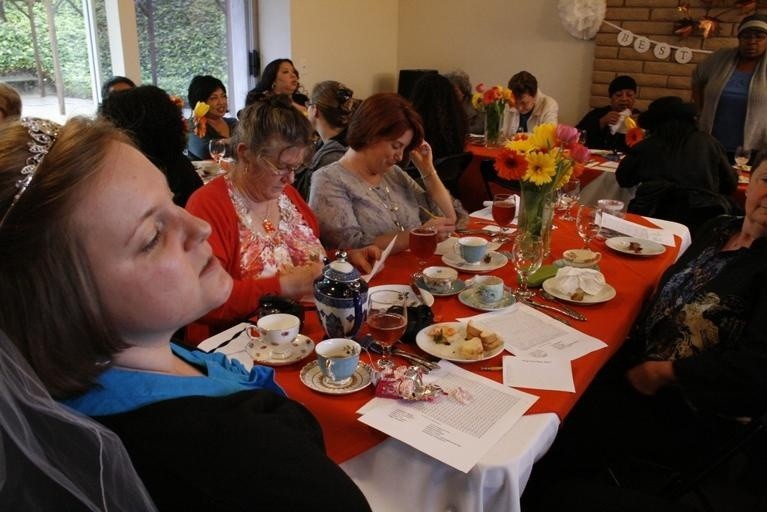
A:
[742,33,764,42]
[261,157,302,175]
[304,101,314,107]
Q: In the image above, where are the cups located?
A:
[576,205,603,250]
[557,178,580,222]
[422,266,457,294]
[245,313,300,358]
[315,338,362,382]
[513,234,543,299]
[491,194,516,244]
[458,236,487,266]
[365,290,407,371]
[473,276,504,305]
[408,221,438,279]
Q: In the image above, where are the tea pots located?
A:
[311,250,368,341]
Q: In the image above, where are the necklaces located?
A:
[246,199,276,234]
[343,154,406,232]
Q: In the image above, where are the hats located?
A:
[608,74,638,98]
[638,95,696,128]
[736,13,767,38]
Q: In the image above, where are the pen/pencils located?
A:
[479,366,502,371]
[418,204,462,238]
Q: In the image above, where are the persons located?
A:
[307,92,469,257]
[692,14,767,164]
[0,117,367,512]
[412,73,469,163]
[444,69,484,134]
[615,95,739,213]
[187,74,240,161]
[291,80,354,204]
[245,58,311,115]
[183,95,381,351]
[575,74,648,150]
[499,69,560,141]
[625,149,767,399]
[97,84,204,209]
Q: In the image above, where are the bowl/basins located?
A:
[598,200,624,216]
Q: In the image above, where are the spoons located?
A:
[539,288,588,321]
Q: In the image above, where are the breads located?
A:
[458,319,504,361]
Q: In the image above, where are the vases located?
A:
[484,111,503,148]
[512,194,558,299]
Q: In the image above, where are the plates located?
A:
[299,359,375,395]
[441,250,508,274]
[542,275,616,306]
[458,289,517,311]
[366,283,421,310]
[604,236,666,258]
[415,278,465,297]
[245,334,315,366]
[416,321,505,363]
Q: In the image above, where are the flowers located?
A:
[166,92,210,139]
[473,82,515,109]
[494,124,594,250]
